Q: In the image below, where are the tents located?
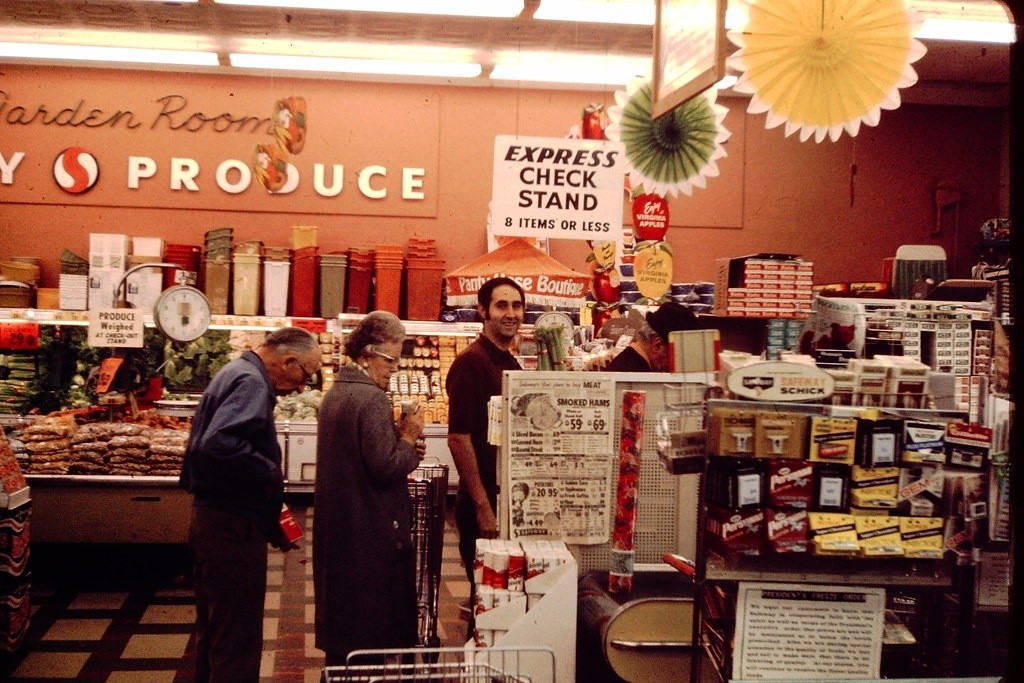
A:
[432,239,591,315]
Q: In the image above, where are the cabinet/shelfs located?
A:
[0,225,812,493]
[689,376,1024,683]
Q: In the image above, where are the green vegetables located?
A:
[0,325,230,413]
[534,325,567,372]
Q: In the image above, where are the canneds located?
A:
[401,401,418,423]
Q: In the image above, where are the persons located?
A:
[316,310,426,683]
[602,303,701,372]
[173,326,322,683]
[446,277,526,643]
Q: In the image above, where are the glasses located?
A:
[295,357,313,383]
[370,348,399,362]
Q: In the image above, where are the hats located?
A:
[646,301,702,349]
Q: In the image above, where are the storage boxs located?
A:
[713,251,817,319]
